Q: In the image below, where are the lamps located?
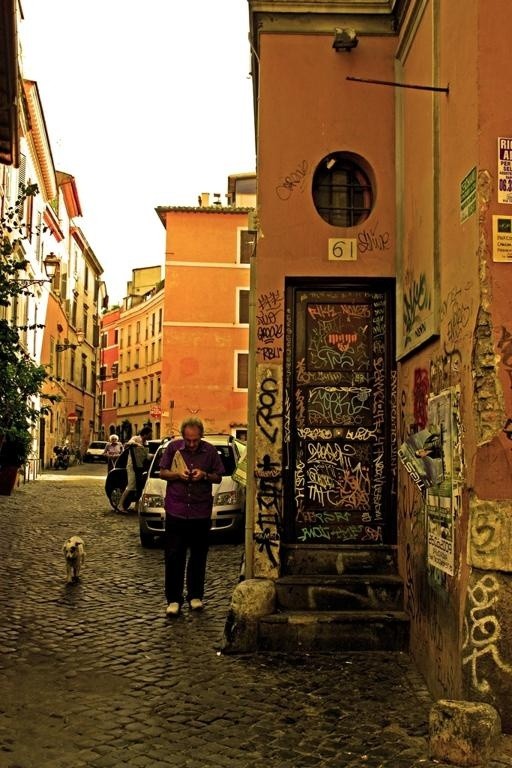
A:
[8,251,60,294]
[55,327,85,352]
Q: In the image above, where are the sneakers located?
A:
[165,600,181,615]
[189,598,206,612]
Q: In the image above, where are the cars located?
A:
[104,439,167,510]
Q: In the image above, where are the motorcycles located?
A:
[53,446,68,470]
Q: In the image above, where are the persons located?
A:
[158,415,226,615]
[102,434,124,474]
[117,427,153,513]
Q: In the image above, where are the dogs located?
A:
[62,534,86,584]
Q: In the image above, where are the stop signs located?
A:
[149,406,161,419]
[67,412,78,424]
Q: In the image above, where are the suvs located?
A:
[138,430,243,547]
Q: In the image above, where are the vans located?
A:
[86,440,108,462]
